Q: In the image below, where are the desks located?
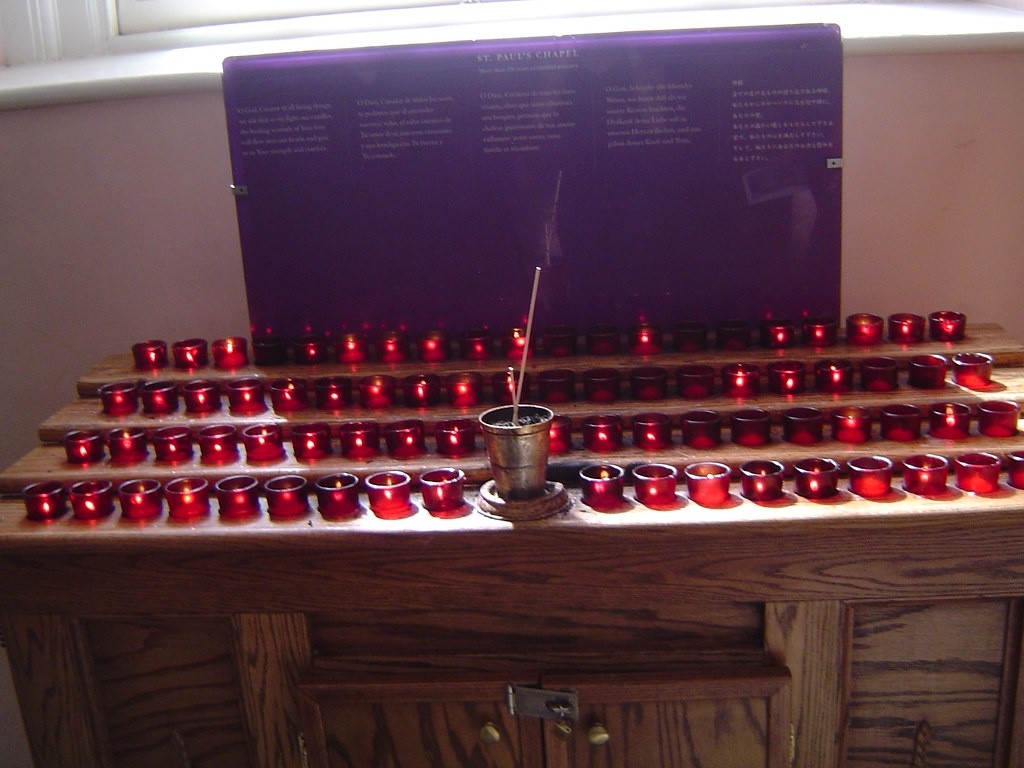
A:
[0,322,1024,768]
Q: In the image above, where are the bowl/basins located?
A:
[23,311,1024,519]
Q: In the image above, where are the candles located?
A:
[141,380,177,409]
[215,475,260,515]
[254,305,1024,522]
[22,479,67,518]
[228,378,264,409]
[172,337,208,367]
[183,378,221,412]
[108,427,148,460]
[211,335,248,369]
[132,340,168,369]
[165,477,210,517]
[153,426,193,459]
[69,478,114,518]
[65,429,105,461]
[119,479,164,517]
[197,424,237,459]
[98,381,139,413]
[242,423,286,463]
